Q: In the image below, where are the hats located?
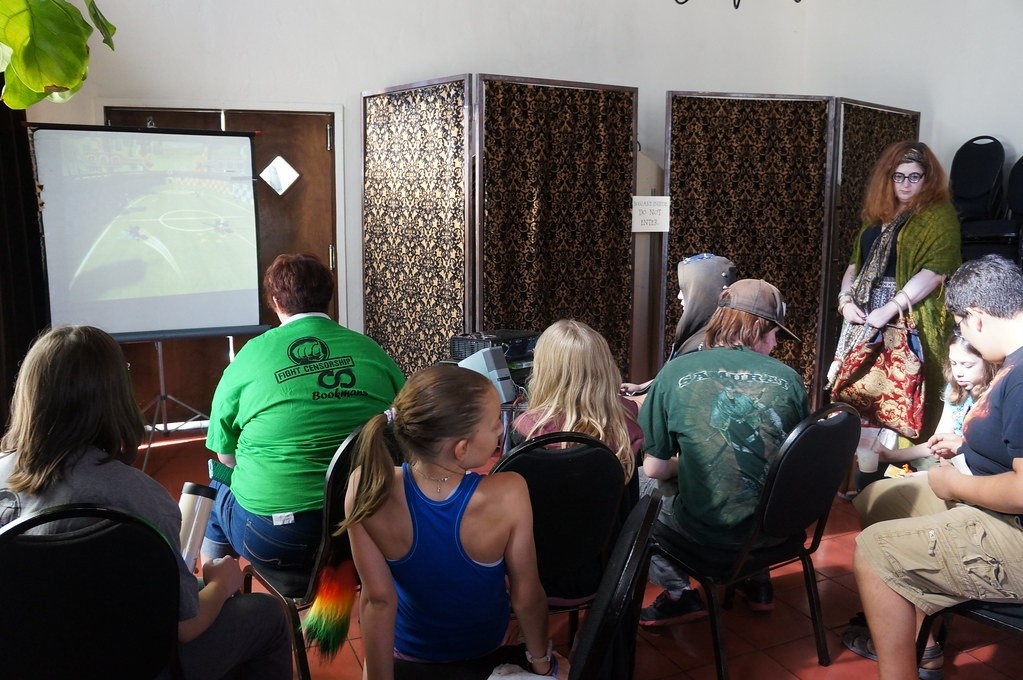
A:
[718,279,803,343]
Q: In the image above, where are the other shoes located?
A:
[837,489,860,501]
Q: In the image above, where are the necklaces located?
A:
[412,465,457,493]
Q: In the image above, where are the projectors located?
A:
[450,329,539,361]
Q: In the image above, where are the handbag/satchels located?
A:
[829,290,925,439]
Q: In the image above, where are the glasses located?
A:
[892,171,926,183]
[952,318,965,337]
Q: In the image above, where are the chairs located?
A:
[948,135,1023,275]
[916,597,1023,680]
[242,411,406,680]
[0,503,184,680]
[487,403,863,680]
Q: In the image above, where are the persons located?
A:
[0,326,294,680]
[618,254,810,626]
[200,256,404,573]
[838,140,962,499]
[840,254,1023,680]
[332,365,572,680]
[510,319,644,608]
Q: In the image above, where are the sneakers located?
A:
[746,576,777,612]
[637,587,710,627]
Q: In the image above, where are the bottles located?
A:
[178,481,217,573]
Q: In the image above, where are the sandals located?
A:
[842,625,944,680]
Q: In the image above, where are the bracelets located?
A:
[541,654,558,677]
[838,290,853,314]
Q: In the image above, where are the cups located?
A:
[856,436,880,473]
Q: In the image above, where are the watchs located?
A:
[525,640,553,662]
